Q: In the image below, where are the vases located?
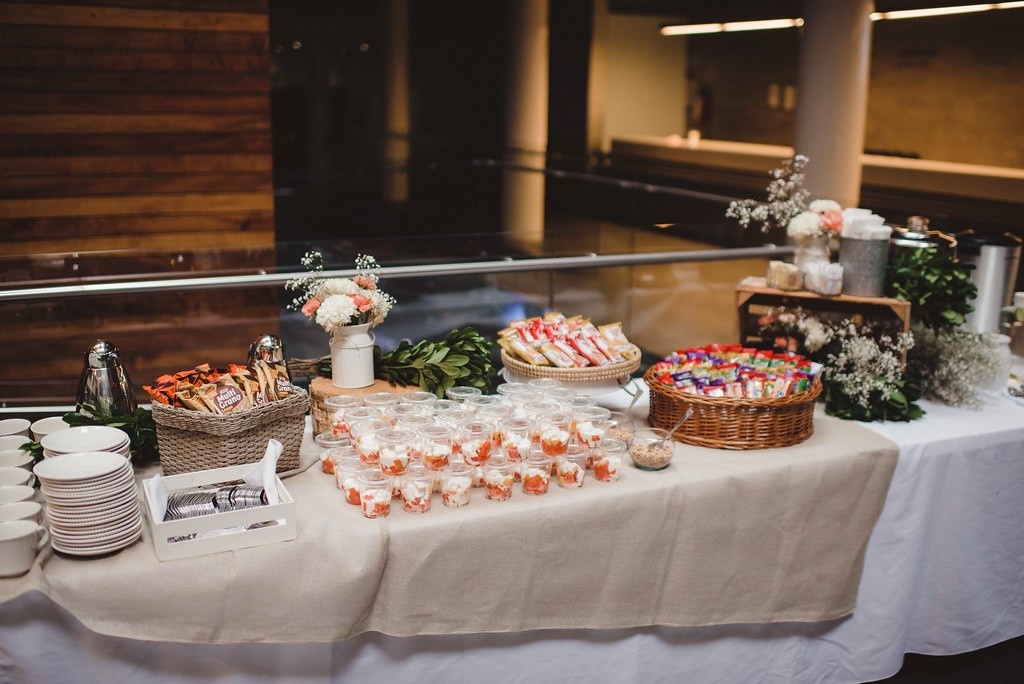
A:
[328,320,376,389]
[793,236,832,275]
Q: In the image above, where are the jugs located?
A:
[76,338,136,417]
[246,333,293,384]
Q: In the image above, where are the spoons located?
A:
[648,408,695,450]
[162,485,266,542]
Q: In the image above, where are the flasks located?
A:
[955,231,1022,333]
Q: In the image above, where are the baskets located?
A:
[500,343,643,384]
[642,349,823,449]
[150,386,310,474]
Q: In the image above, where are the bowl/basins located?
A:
[627,427,676,469]
[600,411,636,451]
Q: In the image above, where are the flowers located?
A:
[753,309,834,358]
[284,248,397,334]
[724,154,845,241]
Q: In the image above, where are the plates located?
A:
[33,453,146,555]
[41,426,134,466]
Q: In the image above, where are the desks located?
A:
[0,352,1024,684]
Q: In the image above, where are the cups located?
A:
[0,520,49,577]
[0,486,35,502]
[31,417,71,443]
[0,467,36,486]
[0,502,46,525]
[839,236,889,297]
[0,450,35,472]
[314,377,625,519]
[0,435,33,450]
[0,419,31,435]
